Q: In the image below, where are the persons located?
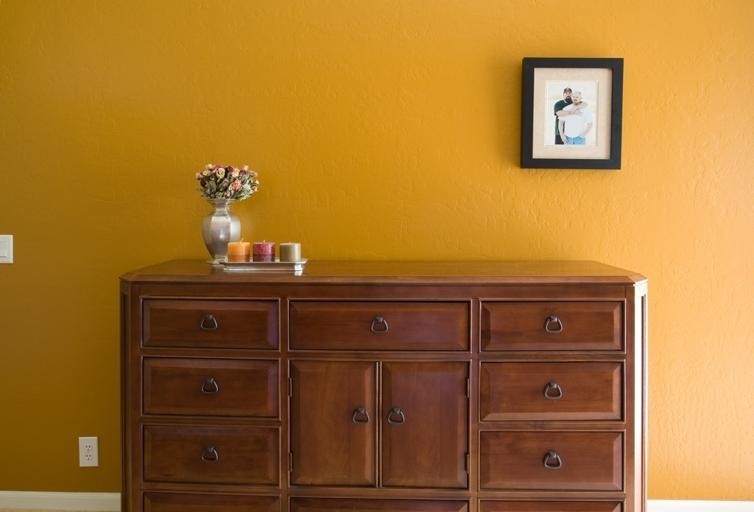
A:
[553,88,593,144]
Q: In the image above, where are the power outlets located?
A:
[78,437,98,468]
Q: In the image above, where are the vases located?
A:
[200,202,241,266]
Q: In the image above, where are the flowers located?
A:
[194,163,260,201]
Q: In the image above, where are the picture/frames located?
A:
[520,57,624,170]
